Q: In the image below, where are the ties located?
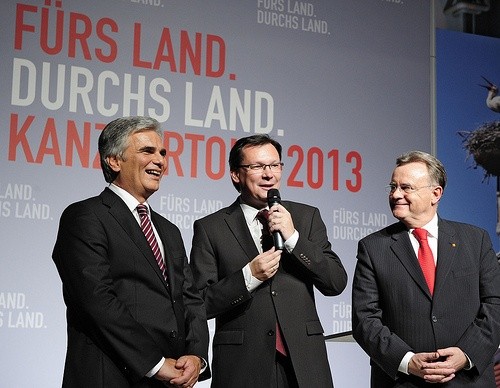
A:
[257,209,288,357]
[412,228,436,298]
[137,203,169,289]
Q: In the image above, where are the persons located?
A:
[189,133,348,388]
[351,151,500,388]
[50,116,212,388]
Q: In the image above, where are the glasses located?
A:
[385,185,438,194]
[238,162,284,174]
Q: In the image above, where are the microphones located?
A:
[267,189,284,251]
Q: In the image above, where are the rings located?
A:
[272,266,276,272]
